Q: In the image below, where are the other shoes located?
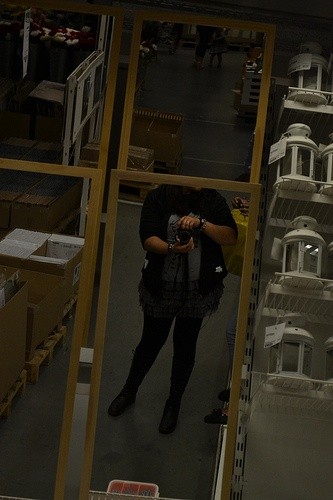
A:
[199,64,205,69]
[208,64,213,68]
[217,64,224,69]
[217,390,230,402]
[204,408,227,424]
[193,61,200,67]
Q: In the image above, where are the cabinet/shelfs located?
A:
[233,99,333,500]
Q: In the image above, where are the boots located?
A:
[160,360,194,433]
[107,355,154,416]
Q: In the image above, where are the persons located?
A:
[10,8,46,39]
[203,197,250,424]
[193,25,227,69]
[107,184,238,435]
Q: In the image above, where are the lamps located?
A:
[282,215,326,290]
[289,42,328,106]
[273,122,318,193]
[265,313,319,390]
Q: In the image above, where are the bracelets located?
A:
[202,223,207,230]
[198,218,206,229]
[168,241,173,253]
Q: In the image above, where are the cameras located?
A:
[177,225,192,245]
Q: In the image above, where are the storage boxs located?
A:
[127,104,188,170]
[0,168,84,402]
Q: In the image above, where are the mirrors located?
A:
[118,9,276,182]
[0,0,123,171]
[79,170,261,500]
[0,156,106,500]
